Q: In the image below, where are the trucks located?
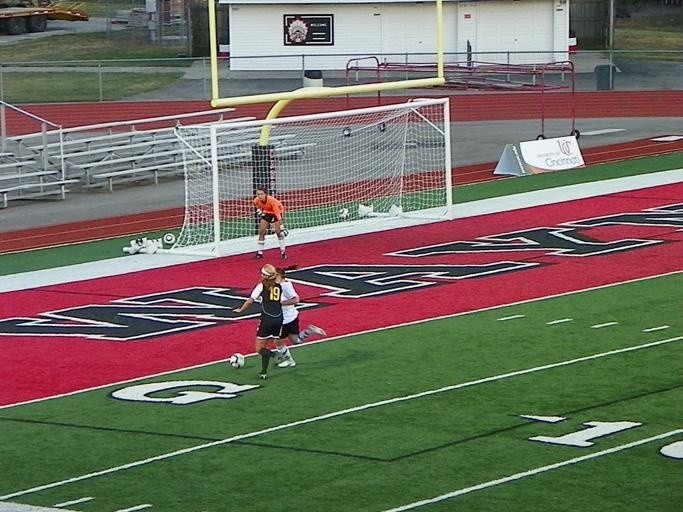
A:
[0,1,89,37]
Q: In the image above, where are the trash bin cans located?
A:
[303,69,324,90]
[595,66,613,90]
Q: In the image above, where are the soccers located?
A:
[337,208,351,220]
[162,232,176,246]
[229,352,245,369]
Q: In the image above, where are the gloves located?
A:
[257,209,262,217]
[280,230,288,238]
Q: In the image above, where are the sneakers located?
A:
[280,250,287,261]
[308,324,327,336]
[258,374,267,380]
[278,361,296,367]
[255,254,264,259]
[273,350,281,368]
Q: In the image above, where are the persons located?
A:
[250,188,288,262]
[232,263,285,380]
[272,263,327,367]
